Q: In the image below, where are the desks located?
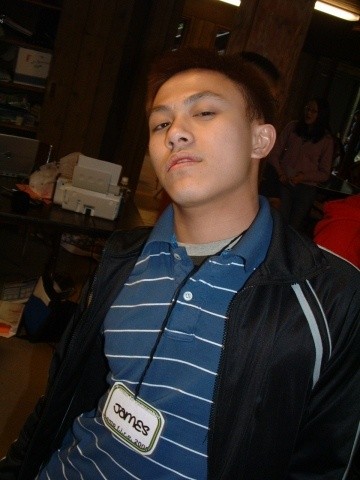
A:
[0,172,145,302]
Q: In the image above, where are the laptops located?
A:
[0,133,40,179]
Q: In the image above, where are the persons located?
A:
[5,46,360,479]
[267,93,334,210]
[225,51,281,210]
